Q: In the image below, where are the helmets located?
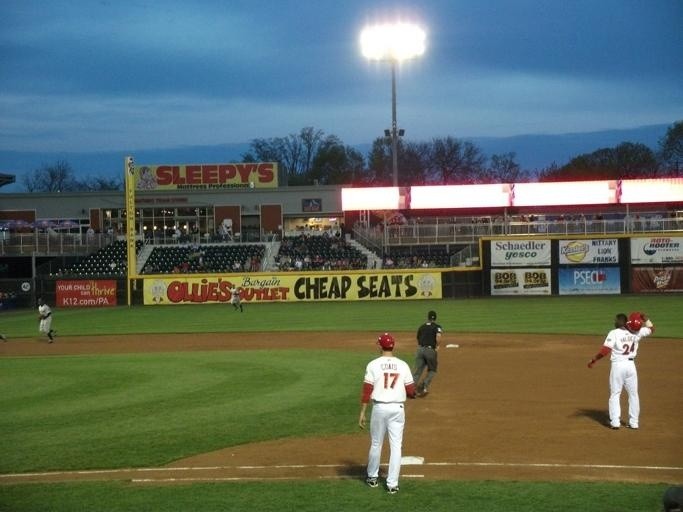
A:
[377,332,395,351]
[626,311,643,332]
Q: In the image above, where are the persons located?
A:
[231,255,261,272]
[226,283,243,312]
[217,221,232,242]
[171,246,205,273]
[376,208,678,239]
[408,311,442,397]
[588,313,656,430]
[86,225,97,246]
[382,256,450,268]
[107,224,114,243]
[38,297,58,342]
[274,222,367,270]
[359,333,416,494]
[192,222,201,243]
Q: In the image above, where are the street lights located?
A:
[357,17,427,186]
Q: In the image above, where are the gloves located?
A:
[587,359,595,368]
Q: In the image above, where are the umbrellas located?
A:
[0,220,79,253]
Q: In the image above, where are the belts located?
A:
[422,345,435,349]
[628,358,633,360]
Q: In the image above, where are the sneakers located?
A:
[366,478,378,488]
[609,424,638,430]
[386,486,400,495]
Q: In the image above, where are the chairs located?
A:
[50,236,450,278]
